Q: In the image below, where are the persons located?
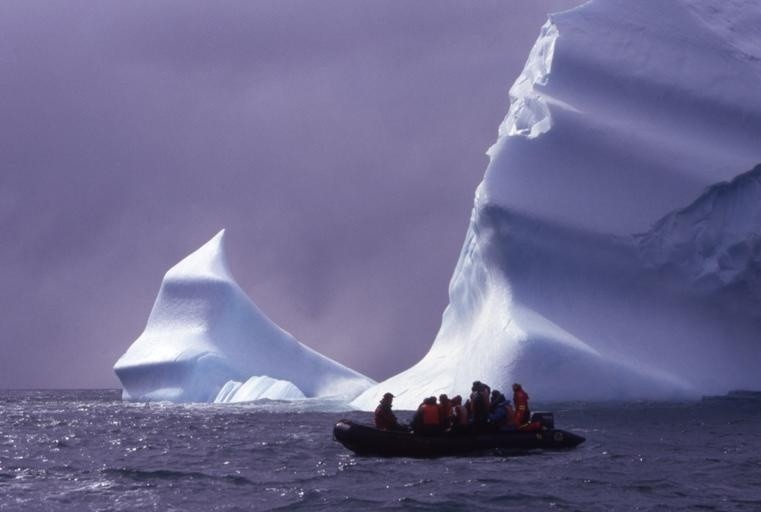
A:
[408,380,513,430]
[375,391,404,430]
[511,382,532,426]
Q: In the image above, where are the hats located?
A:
[383,392,396,399]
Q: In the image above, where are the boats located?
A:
[334,419,585,458]
